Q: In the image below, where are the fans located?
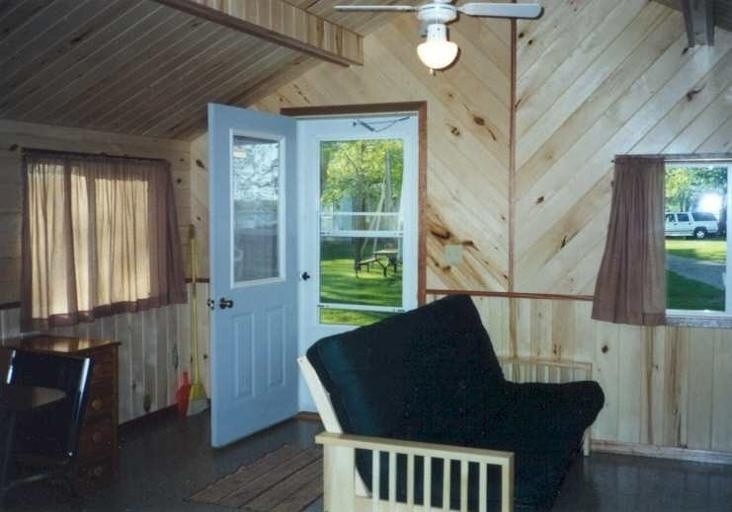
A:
[334,0,543,36]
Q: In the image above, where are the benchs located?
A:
[295,293,604,510]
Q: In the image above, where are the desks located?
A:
[0,383,66,483]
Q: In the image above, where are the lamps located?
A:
[414,22,460,75]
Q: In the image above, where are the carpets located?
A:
[183,441,323,511]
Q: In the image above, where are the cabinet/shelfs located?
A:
[0,332,124,498]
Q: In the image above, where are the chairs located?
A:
[0,349,92,493]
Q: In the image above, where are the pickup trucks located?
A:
[664,212,720,239]
